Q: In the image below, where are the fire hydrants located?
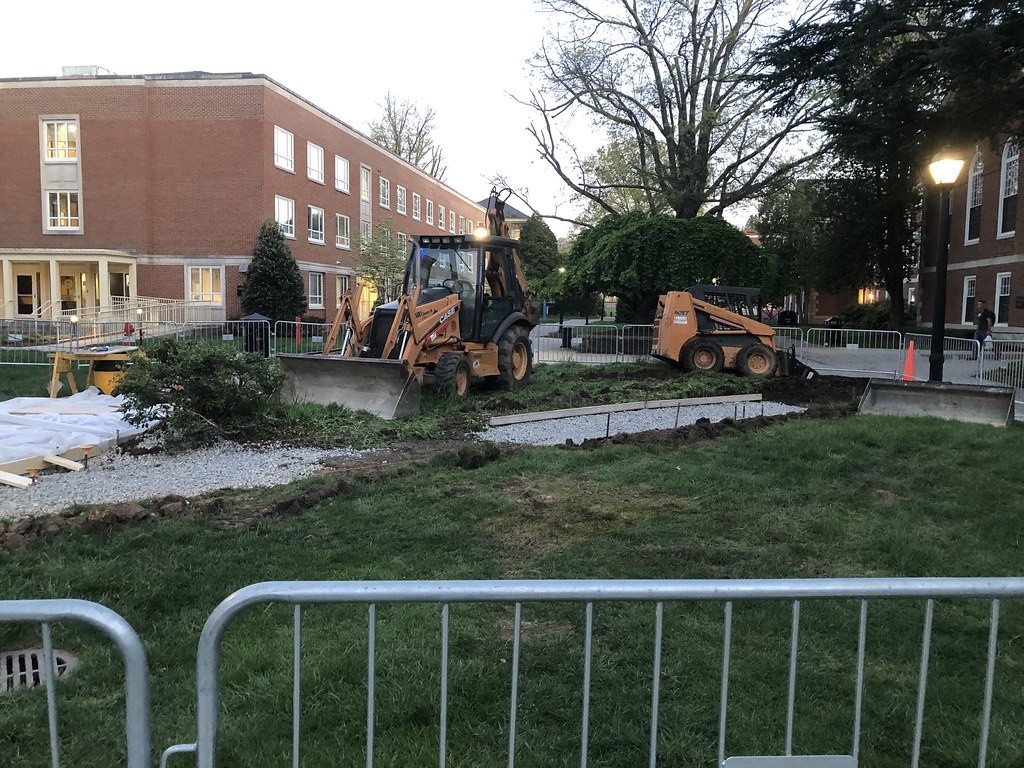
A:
[121,323,135,348]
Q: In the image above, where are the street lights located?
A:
[928,144,966,382]
[557,268,566,334]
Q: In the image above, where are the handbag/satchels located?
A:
[983,335,993,351]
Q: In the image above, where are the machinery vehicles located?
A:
[262,184,544,422]
[651,283,822,383]
[44,348,160,397]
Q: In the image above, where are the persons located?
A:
[964,300,1002,361]
[340,288,354,325]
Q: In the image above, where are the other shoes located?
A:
[994,353,1001,360]
[967,357,977,360]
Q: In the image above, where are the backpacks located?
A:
[983,308,995,326]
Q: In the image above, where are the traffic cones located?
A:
[901,339,918,380]
